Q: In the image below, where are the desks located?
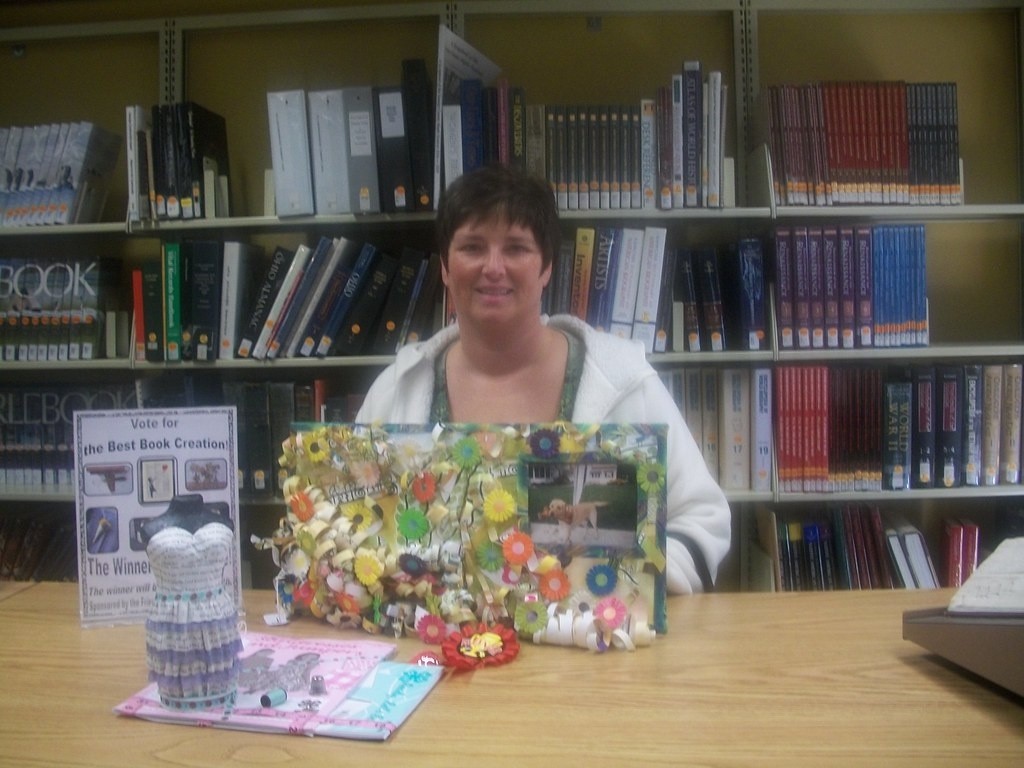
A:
[0,582,1024,768]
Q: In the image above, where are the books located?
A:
[1,25,1024,591]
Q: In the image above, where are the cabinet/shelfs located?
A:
[1,1,1023,586]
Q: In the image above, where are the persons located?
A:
[350,168,733,594]
